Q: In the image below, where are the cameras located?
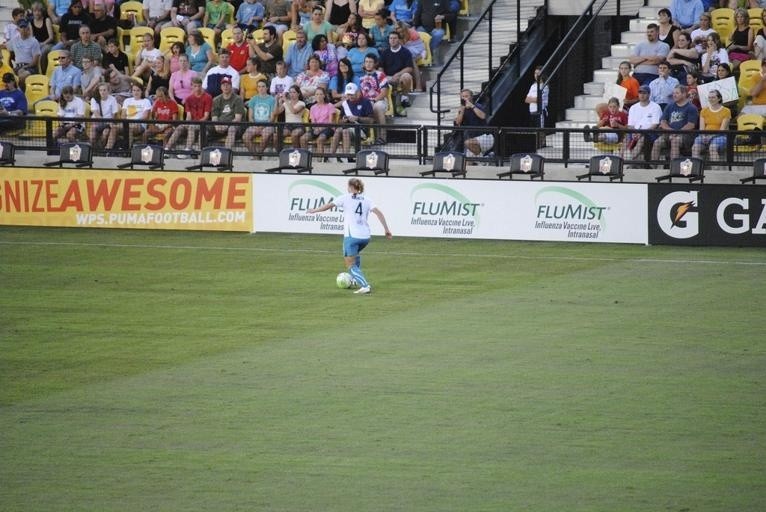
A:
[246,33,253,39]
[279,93,283,98]
[461,99,465,107]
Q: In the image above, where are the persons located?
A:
[306,179,392,295]
[583,0,766,169]
[0,0,461,166]
[525,65,549,149]
[454,89,499,167]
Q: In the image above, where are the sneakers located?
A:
[353,285,371,293]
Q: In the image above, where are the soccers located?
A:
[336,272,353,288]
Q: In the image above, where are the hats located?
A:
[17,19,28,27]
[345,82,357,95]
[220,76,231,83]
[638,84,650,92]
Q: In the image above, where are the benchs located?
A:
[520,0,766,185]
[0,0,544,182]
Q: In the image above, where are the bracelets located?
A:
[471,105,476,109]
